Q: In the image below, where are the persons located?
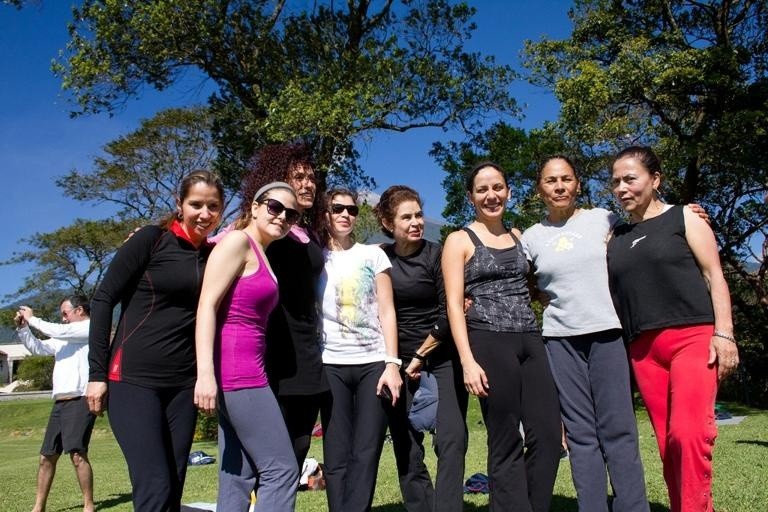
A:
[86,171,226,512]
[376,185,472,512]
[606,146,740,512]
[313,189,402,511]
[256,143,325,499]
[194,181,300,512]
[441,162,562,511]
[520,154,649,511]
[14,295,97,512]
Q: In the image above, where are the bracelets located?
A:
[411,353,424,360]
[713,333,736,343]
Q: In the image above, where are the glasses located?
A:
[333,203,359,217]
[258,198,301,225]
[62,309,74,316]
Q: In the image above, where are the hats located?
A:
[410,370,437,434]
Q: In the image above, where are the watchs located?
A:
[384,358,402,370]
